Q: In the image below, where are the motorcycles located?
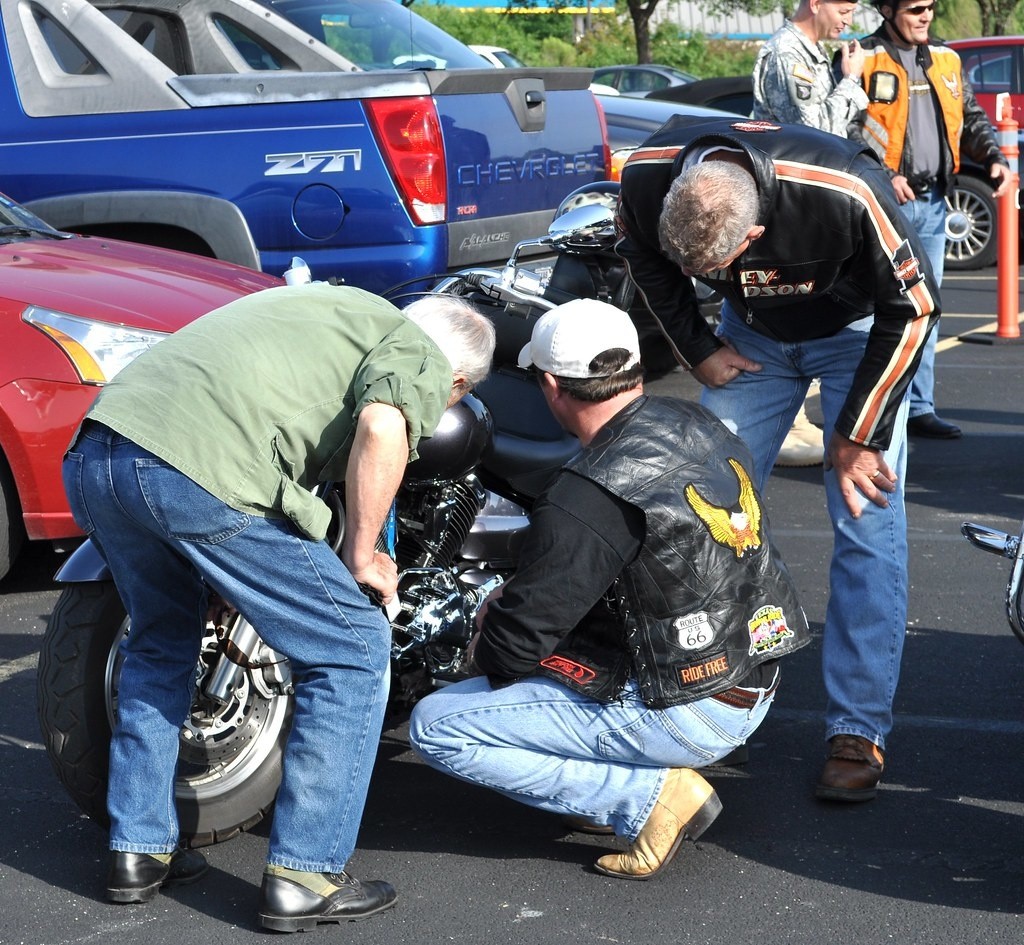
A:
[36,170,676,840]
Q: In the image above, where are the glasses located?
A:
[893,0,936,15]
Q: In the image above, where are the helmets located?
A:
[870,0,900,21]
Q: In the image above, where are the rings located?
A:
[867,470,881,480]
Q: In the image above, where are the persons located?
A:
[622,116,944,808]
[407,301,815,884]
[848,0,1010,445]
[754,0,870,150]
[68,291,494,936]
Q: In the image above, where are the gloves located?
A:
[905,169,932,205]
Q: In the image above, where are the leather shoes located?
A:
[905,411,963,438]
[569,825,616,832]
[594,767,723,880]
[257,862,399,932]
[817,734,884,799]
[107,843,209,902]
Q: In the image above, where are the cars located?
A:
[237,0,1024,267]
[1,191,292,595]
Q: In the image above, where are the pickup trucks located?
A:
[1,0,629,318]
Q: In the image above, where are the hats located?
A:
[515,298,641,378]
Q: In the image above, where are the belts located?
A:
[711,663,783,708]
